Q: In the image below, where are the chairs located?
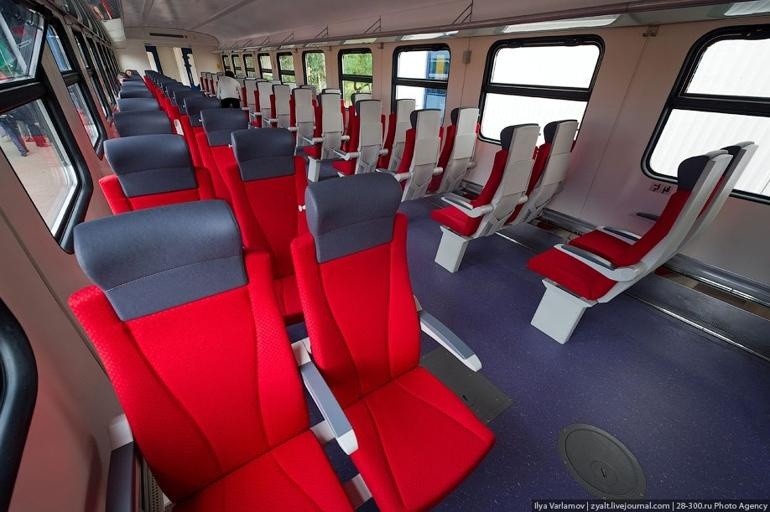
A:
[65,68,762,512]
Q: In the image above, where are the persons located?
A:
[215,70,244,109]
[125,69,135,77]
[116,71,129,85]
